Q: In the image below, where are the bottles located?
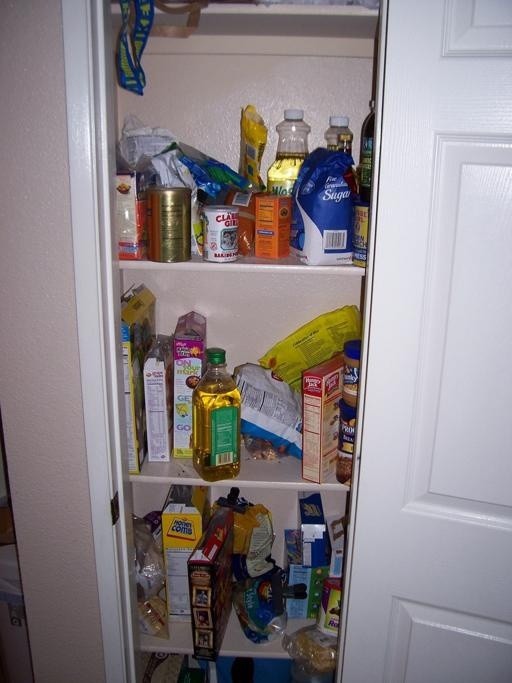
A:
[191,347,241,482]
[267,100,375,198]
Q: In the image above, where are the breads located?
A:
[295,631,336,671]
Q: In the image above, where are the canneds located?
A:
[204,205,240,262]
